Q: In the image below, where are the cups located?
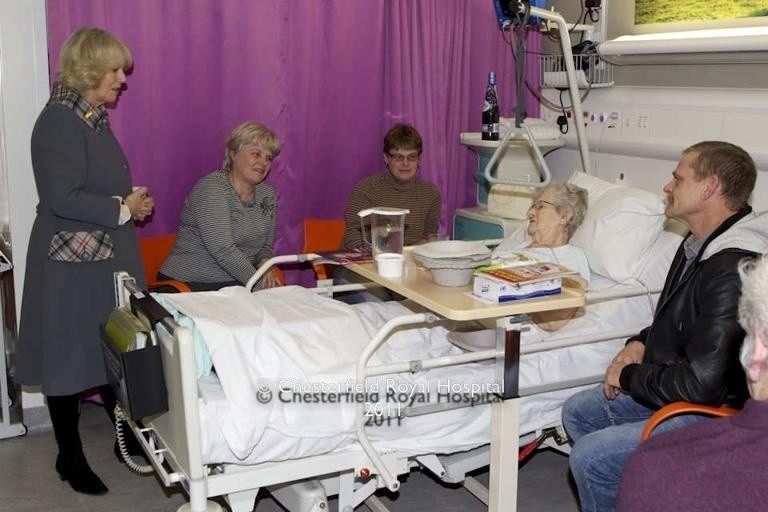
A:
[356,207,410,278]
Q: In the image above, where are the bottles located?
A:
[482,72,499,141]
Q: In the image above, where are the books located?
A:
[103,306,157,352]
[471,257,581,289]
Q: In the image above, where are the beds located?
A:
[113,169,690,512]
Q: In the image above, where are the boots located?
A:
[47,384,149,496]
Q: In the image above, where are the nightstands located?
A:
[452,207,526,247]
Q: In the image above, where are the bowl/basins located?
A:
[430,268,474,287]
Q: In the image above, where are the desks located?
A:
[459,131,565,220]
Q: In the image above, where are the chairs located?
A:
[303,218,346,282]
[138,232,190,293]
[641,402,741,443]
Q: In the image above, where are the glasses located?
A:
[532,200,553,210]
[388,152,419,162]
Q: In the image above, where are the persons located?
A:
[16,25,153,496]
[560,141,768,510]
[489,181,589,333]
[611,252,768,512]
[154,118,282,292]
[331,121,442,305]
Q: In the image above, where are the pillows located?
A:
[564,170,670,286]
